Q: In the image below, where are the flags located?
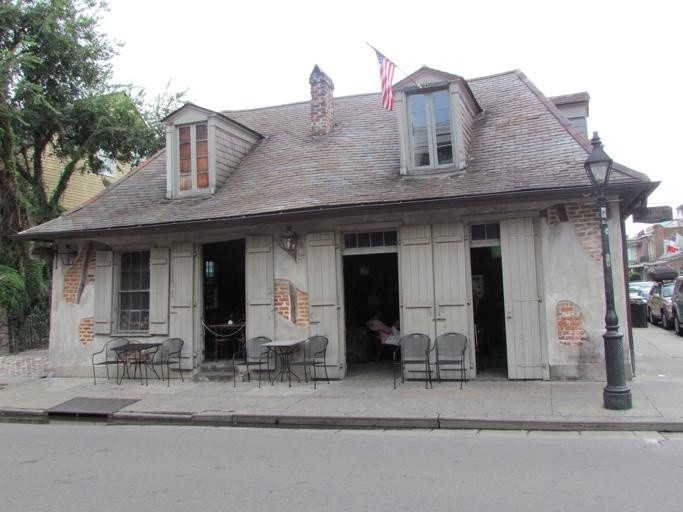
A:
[374,50,398,111]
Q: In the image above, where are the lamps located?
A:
[278,222,298,253]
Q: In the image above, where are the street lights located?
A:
[583,131,632,411]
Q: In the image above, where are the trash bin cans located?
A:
[630,296,648,329]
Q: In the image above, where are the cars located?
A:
[628,277,683,335]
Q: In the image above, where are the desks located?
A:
[209,322,244,360]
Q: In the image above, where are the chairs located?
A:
[389,331,468,390]
[91,336,184,386]
[231,334,330,390]
[367,320,400,364]
